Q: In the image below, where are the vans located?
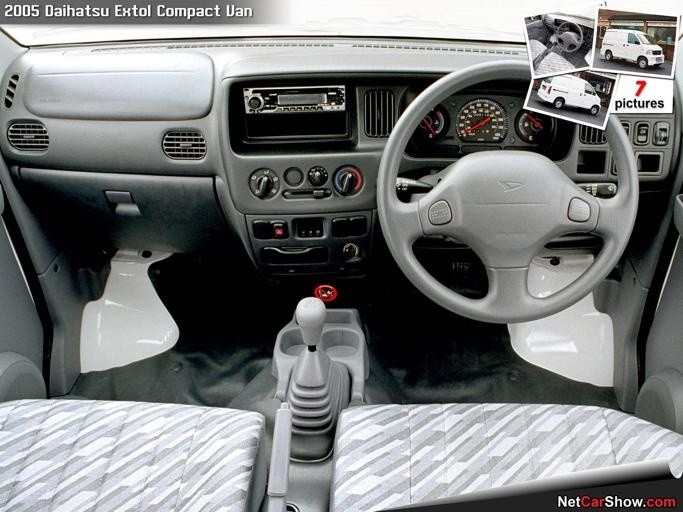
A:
[536,73,603,116]
[597,26,666,70]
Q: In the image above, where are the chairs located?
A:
[535,52,575,74]
[530,39,547,65]
